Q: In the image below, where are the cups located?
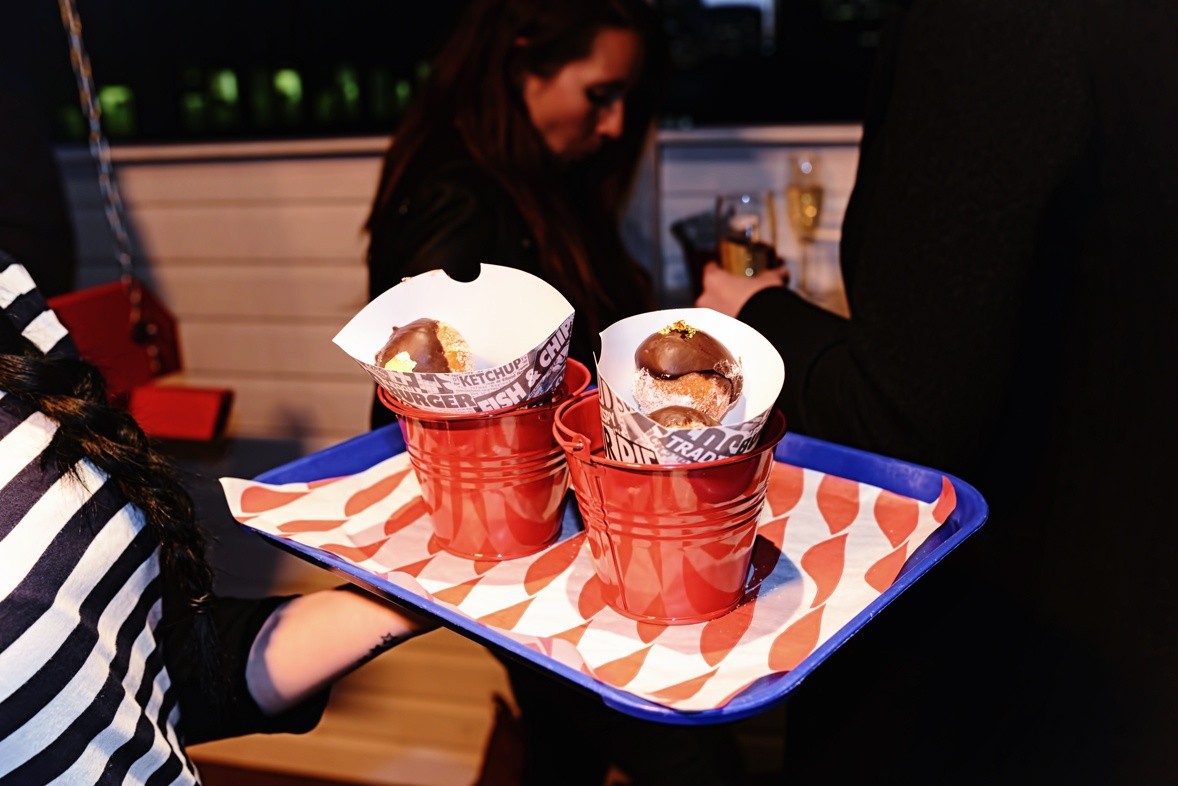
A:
[552,384,784,624]
[713,188,776,282]
[376,354,594,561]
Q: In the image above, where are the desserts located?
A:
[375,317,473,373]
[635,320,744,429]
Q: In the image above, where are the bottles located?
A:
[784,156,826,303]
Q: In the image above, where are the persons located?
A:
[695,0,1178,785]
[1,234,441,785]
[365,0,748,786]
